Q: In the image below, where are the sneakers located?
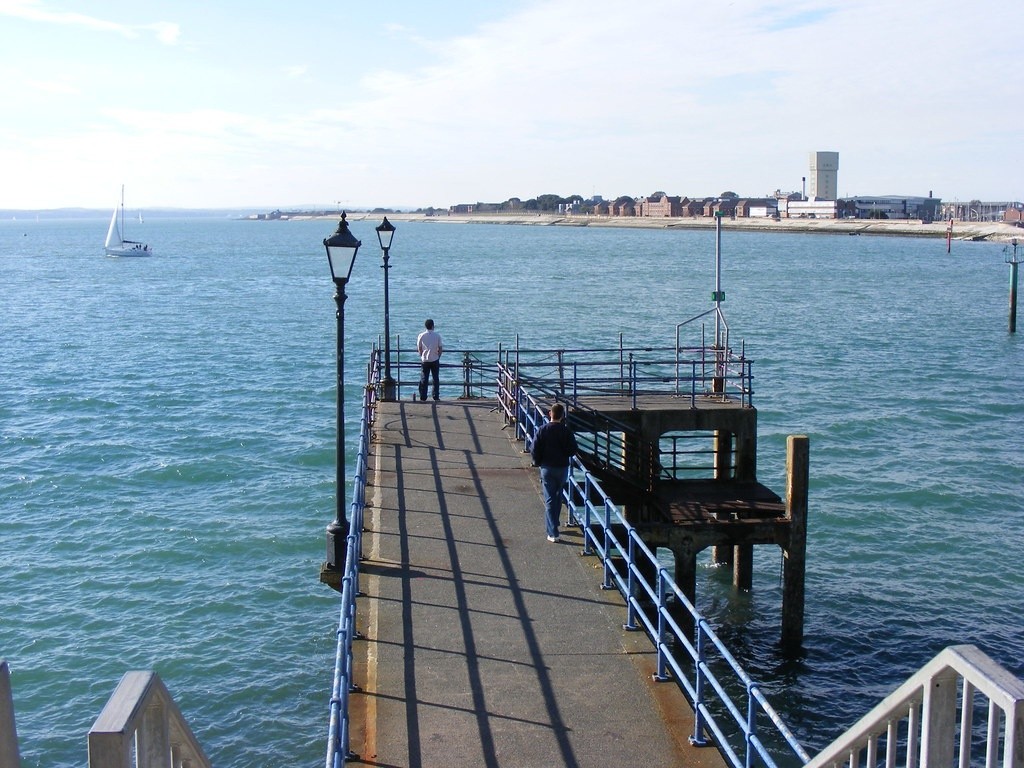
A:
[420,399,427,403]
[547,535,559,542]
[434,399,440,403]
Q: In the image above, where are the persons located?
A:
[530,404,579,543]
[417,319,443,403]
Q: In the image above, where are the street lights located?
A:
[321,209,362,572]
[373,216,399,403]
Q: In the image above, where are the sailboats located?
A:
[102,184,153,258]
